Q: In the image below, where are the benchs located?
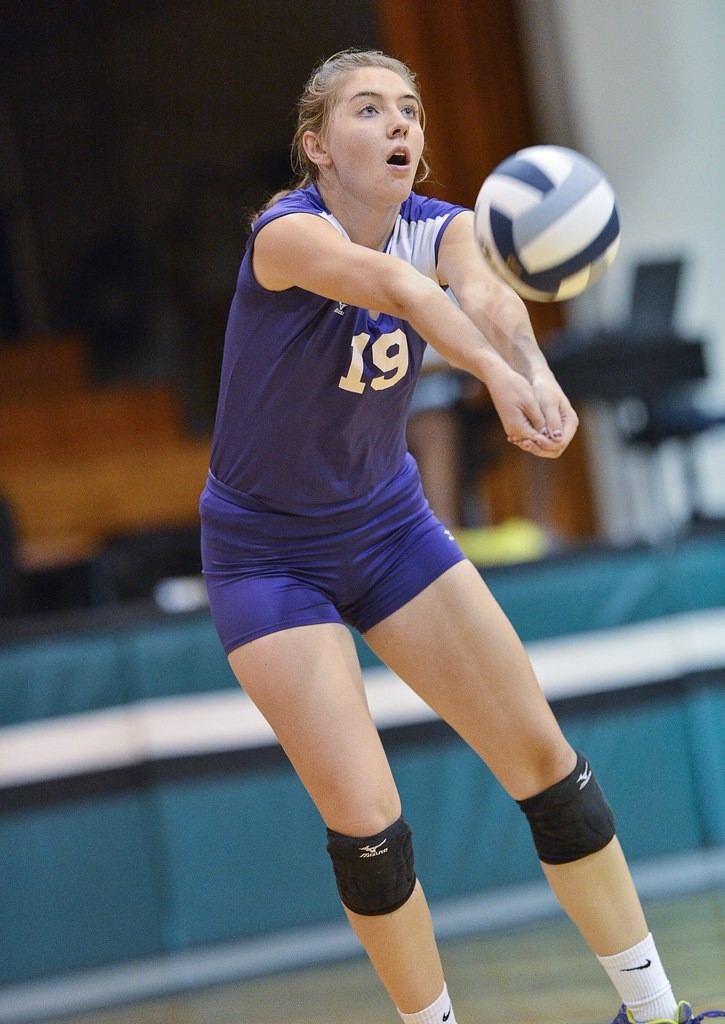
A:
[1,341,216,566]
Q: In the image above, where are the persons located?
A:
[199,51,725,1024]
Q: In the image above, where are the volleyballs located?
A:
[471,141,623,304]
